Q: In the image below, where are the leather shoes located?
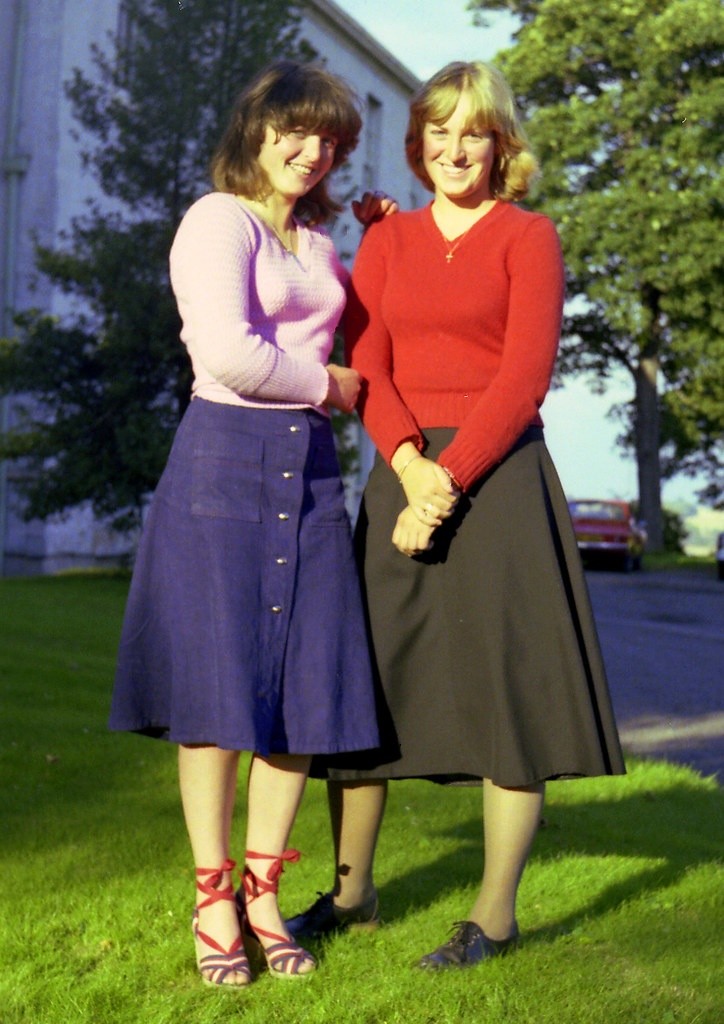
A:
[418,920,520,971]
[284,888,381,944]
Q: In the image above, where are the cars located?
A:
[717,533,724,580]
[568,499,648,573]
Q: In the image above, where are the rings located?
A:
[423,504,432,513]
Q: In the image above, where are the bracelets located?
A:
[400,454,422,480]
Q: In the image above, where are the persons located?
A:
[285,63,627,976]
[107,63,399,987]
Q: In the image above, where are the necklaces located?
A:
[254,203,293,252]
[439,203,488,264]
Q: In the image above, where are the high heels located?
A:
[191,848,318,989]
